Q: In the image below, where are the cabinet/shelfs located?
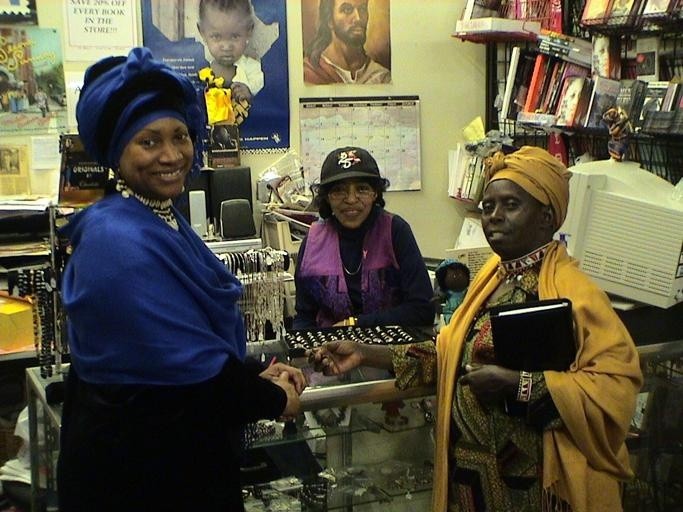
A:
[25,327,435,512]
[485,0,683,344]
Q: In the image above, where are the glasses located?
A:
[322,186,378,199]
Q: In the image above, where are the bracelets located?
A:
[516,370,534,403]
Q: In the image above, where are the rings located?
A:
[286,325,414,350]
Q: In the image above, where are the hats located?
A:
[317,146,381,184]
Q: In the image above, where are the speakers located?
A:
[220,198,256,238]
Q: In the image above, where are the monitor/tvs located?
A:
[552,161,683,311]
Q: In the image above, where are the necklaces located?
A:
[216,247,287,342]
[343,262,361,275]
[17,267,68,379]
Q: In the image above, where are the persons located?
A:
[0,79,50,117]
[196,0,265,104]
[287,145,435,392]
[53,44,309,512]
[304,144,645,512]
[303,0,391,85]
